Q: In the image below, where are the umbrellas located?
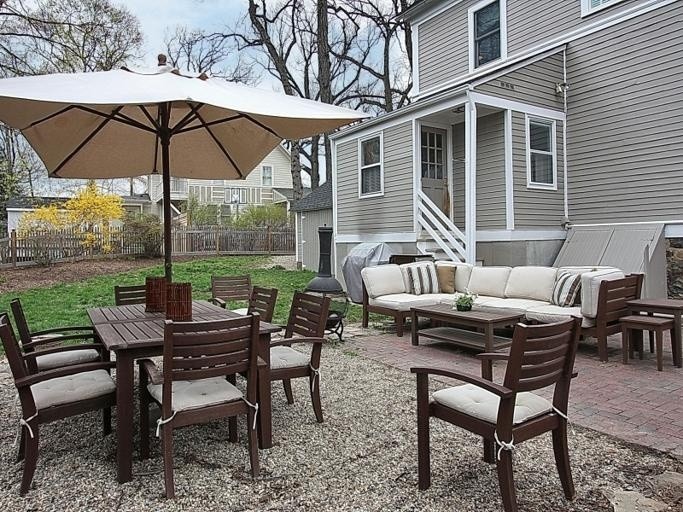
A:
[0,55,373,283]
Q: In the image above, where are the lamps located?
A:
[554,80,570,94]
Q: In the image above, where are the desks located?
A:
[621,297,683,368]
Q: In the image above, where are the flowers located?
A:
[451,288,480,305]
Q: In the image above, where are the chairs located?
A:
[406,314,587,510]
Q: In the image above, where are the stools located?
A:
[617,313,674,370]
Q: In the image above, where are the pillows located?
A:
[406,264,443,295]
[435,264,458,293]
[547,268,584,308]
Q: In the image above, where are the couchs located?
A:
[359,255,645,364]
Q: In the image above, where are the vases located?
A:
[457,303,472,310]
